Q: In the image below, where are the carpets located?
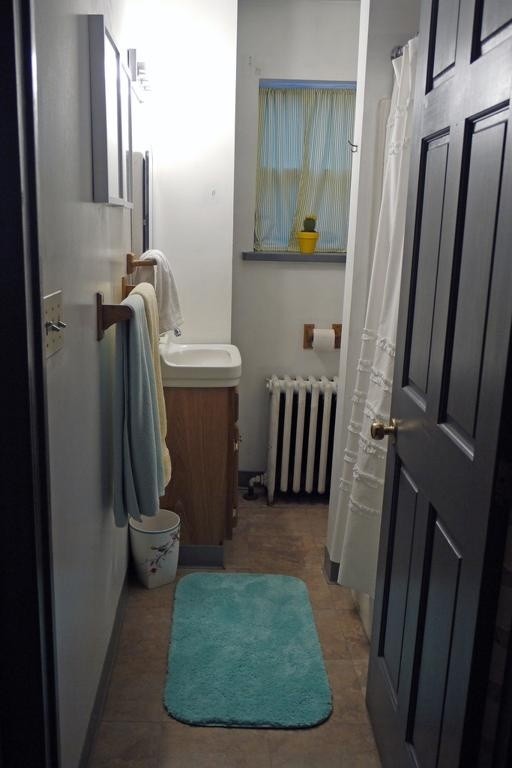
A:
[164,571,334,729]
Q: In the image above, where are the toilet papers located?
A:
[311,327,335,350]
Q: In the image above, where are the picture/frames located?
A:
[88,14,133,209]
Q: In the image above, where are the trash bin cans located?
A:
[129,509,182,589]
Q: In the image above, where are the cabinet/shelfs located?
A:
[159,387,240,567]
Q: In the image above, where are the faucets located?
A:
[174,326,182,338]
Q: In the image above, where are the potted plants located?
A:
[296,215,320,254]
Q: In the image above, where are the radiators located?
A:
[263,375,337,506]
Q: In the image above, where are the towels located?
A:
[134,249,184,338]
[112,282,174,527]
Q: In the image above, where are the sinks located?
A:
[158,331,241,387]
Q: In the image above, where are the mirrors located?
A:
[131,92,153,260]
